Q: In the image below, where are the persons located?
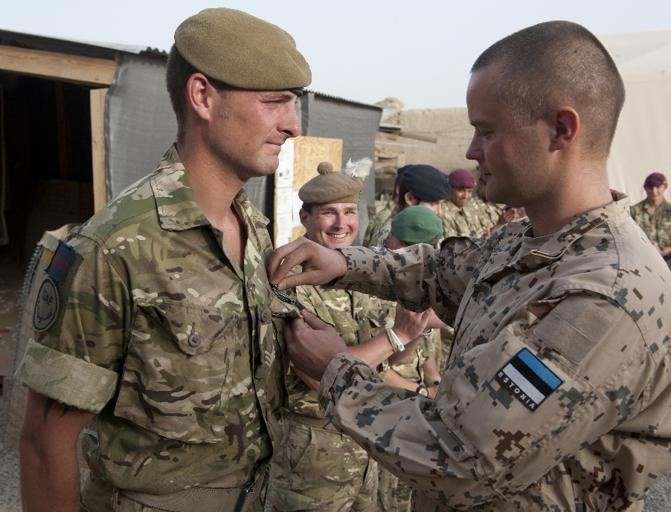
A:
[13,7,311,512]
[260,20,671,512]
[272,163,432,511]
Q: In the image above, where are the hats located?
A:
[391,205,442,243]
[299,162,363,205]
[448,169,477,188]
[397,165,450,201]
[173,8,312,91]
[645,173,665,188]
